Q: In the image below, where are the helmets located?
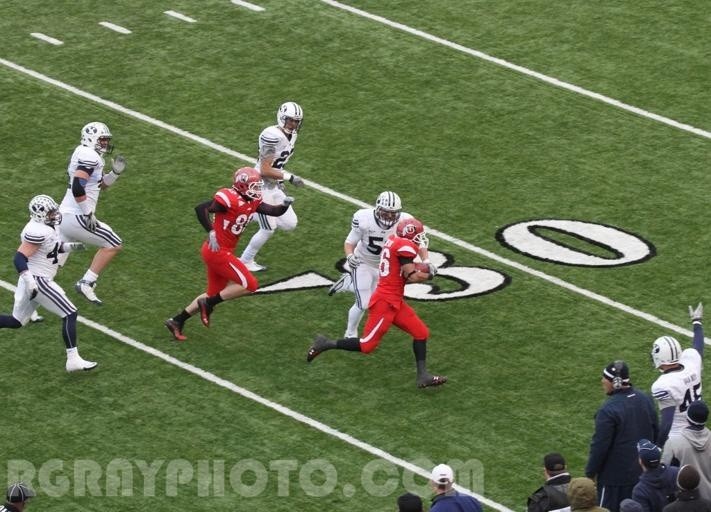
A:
[80,122,114,155]
[652,336,683,368]
[396,217,427,248]
[375,191,402,226]
[276,102,303,135]
[29,194,63,226]
[232,167,264,202]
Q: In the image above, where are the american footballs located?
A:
[415,262,437,277]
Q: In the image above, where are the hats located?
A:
[637,439,662,466]
[687,400,709,426]
[677,464,701,491]
[544,453,565,470]
[430,464,453,485]
[603,361,631,386]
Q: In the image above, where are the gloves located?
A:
[64,242,87,253]
[688,301,704,326]
[84,212,100,233]
[281,196,295,208]
[426,262,438,280]
[289,175,305,187]
[25,279,38,301]
[347,255,361,269]
[111,155,128,174]
[207,230,221,252]
[275,180,287,193]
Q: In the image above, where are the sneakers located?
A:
[197,297,214,327]
[244,261,266,272]
[415,375,447,388]
[165,318,188,340]
[307,333,325,363]
[328,274,351,296]
[75,280,102,304]
[66,357,97,372]
[29,309,43,322]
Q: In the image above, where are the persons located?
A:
[161,164,296,340]
[396,491,423,511]
[327,188,437,338]
[0,483,35,511]
[428,462,482,511]
[307,219,447,389]
[526,302,711,512]
[0,194,101,367]
[56,121,126,306]
[238,101,304,273]
